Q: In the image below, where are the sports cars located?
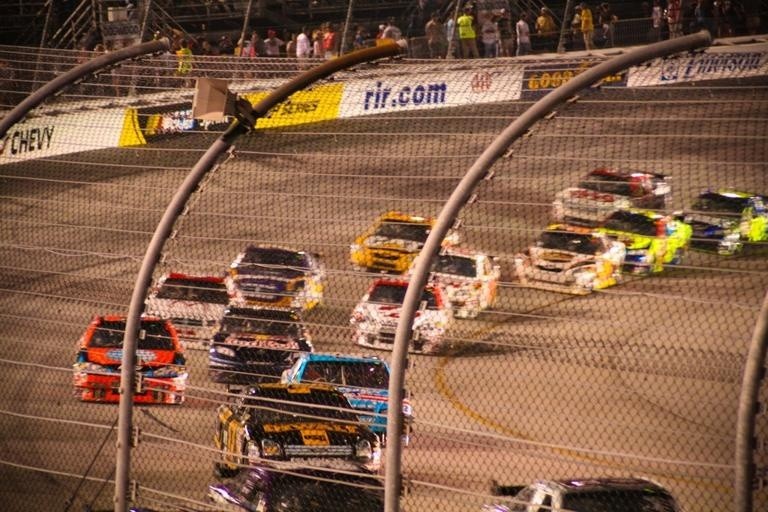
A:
[516,167,767,297]
[485,478,672,511]
[348,211,501,354]
[211,353,413,511]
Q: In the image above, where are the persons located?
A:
[218,17,402,58]
[426,8,514,58]
[516,7,555,56]
[75,32,208,88]
[571,0,618,51]
[652,1,766,40]
